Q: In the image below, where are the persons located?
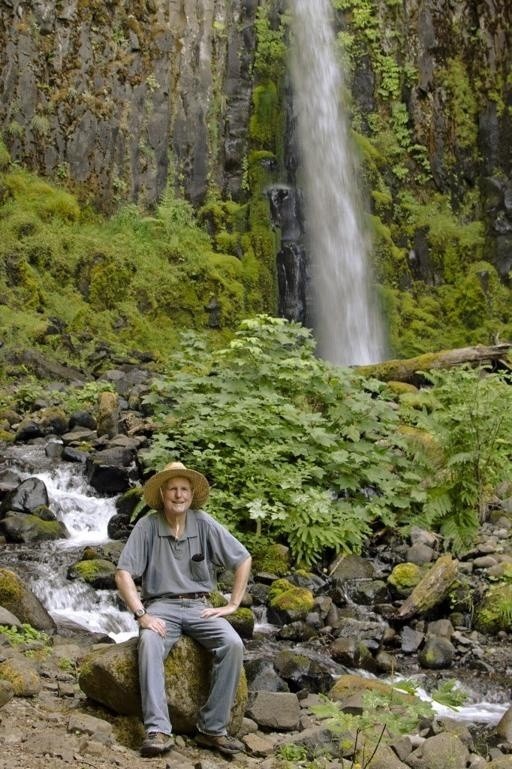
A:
[114,462,252,755]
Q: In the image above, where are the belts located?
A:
[154,592,211,600]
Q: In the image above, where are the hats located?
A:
[142,461,209,510]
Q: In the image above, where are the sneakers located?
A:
[195,734,244,755]
[140,733,174,755]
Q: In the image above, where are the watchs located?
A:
[134,608,147,620]
